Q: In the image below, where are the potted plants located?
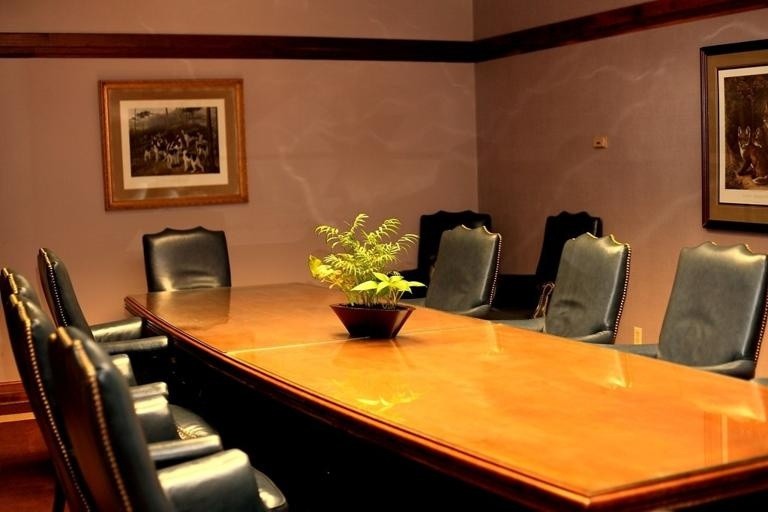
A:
[307,214,428,338]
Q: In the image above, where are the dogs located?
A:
[145,130,208,173]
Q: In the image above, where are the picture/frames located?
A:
[98,78,248,211]
[700,39,768,232]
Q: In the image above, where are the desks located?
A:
[123,282,768,512]
